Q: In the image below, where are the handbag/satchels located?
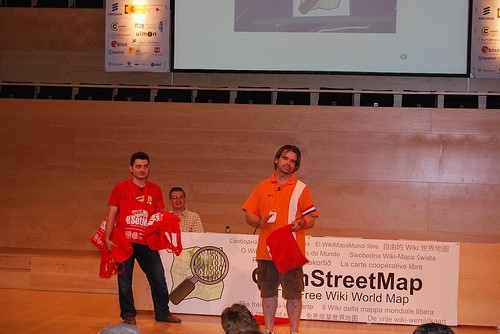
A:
[91,214,133,278]
[142,210,183,256]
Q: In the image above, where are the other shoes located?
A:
[165,313,181,323]
[123,316,136,325]
[262,329,276,334]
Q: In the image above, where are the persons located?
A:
[168,186,204,232]
[220,303,265,334]
[105,152,182,323]
[240,144,319,334]
[413,322,455,334]
[97,323,139,334]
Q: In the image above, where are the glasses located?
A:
[173,196,183,199]
